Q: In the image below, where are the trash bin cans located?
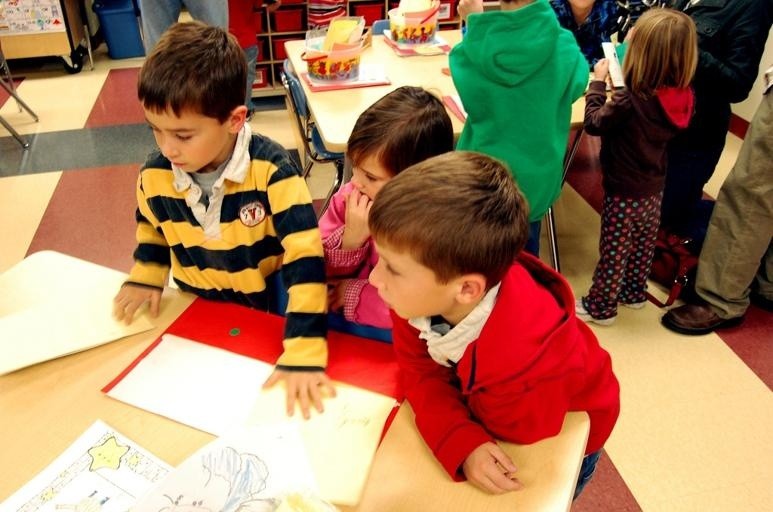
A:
[92,0,146,59]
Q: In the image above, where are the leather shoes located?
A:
[661,299,745,337]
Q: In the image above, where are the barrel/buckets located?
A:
[300,27,365,86]
[387,0,442,47]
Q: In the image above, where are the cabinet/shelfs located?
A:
[251,0,501,99]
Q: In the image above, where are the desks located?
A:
[0,249,591,512]
[282,28,614,275]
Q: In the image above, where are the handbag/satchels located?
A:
[645,226,699,308]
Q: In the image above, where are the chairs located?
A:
[372,19,390,33]
[0,41,40,151]
[280,59,346,223]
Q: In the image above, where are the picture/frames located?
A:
[251,67,268,88]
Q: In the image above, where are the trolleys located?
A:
[0,0,100,77]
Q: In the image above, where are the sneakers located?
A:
[574,296,646,327]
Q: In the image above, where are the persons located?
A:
[368,150,620,502]
[553,0,621,72]
[113,23,337,419]
[449,0,589,261]
[228,0,274,121]
[610,2,772,274]
[138,0,228,61]
[571,7,698,325]
[662,66,772,334]
[319,86,453,331]
